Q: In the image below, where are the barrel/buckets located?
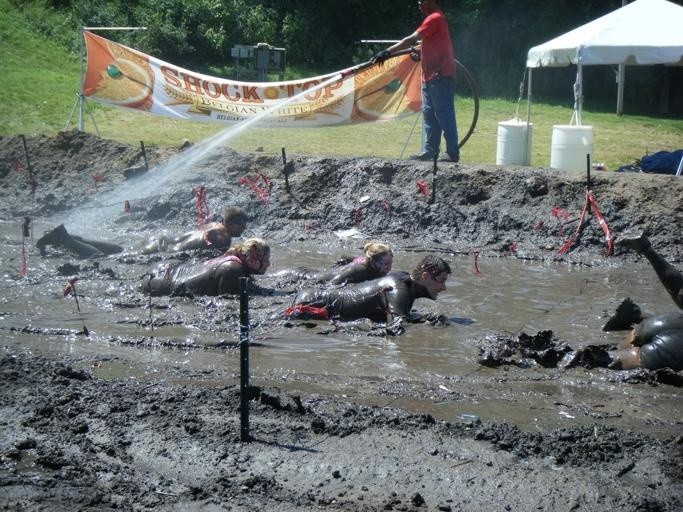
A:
[496,120,533,167]
[551,123,595,176]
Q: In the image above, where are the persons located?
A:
[271,254,453,330]
[598,308,682,374]
[65,238,288,302]
[602,229,683,332]
[34,205,246,261]
[366,0,460,162]
[271,241,395,286]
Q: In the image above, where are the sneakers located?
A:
[437,152,460,163]
[34,221,69,252]
[615,228,652,255]
[601,297,641,334]
[406,151,438,161]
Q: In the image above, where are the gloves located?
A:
[373,47,390,66]
[408,52,421,64]
